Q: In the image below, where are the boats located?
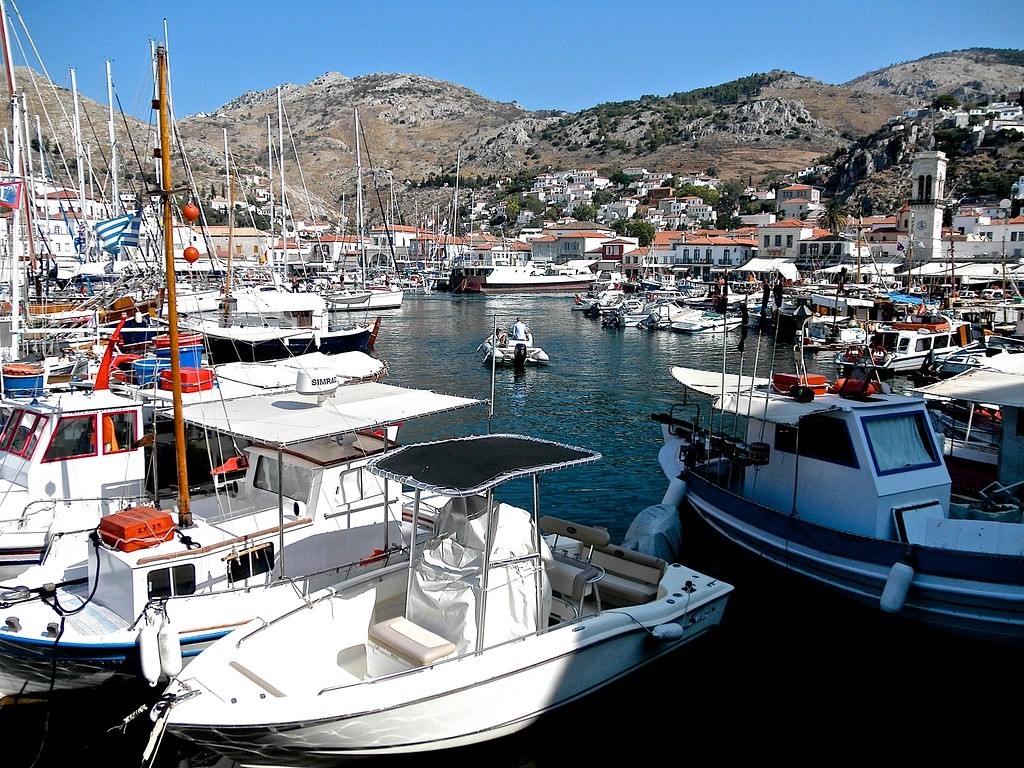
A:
[483,327,549,368]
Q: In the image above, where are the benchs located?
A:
[366,615,456,679]
[539,514,610,621]
[580,542,668,608]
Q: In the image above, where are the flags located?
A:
[0,181,23,209]
[95,209,142,254]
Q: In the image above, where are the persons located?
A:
[290,276,300,293]
[485,328,509,344]
[512,316,526,341]
[383,270,391,290]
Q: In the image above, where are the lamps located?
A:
[335,432,358,452]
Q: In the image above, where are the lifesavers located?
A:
[922,285,927,292]
[112,353,142,380]
[845,344,863,364]
[645,292,659,300]
[892,282,898,290]
[871,345,888,363]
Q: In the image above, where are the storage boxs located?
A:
[161,367,213,393]
[99,506,175,553]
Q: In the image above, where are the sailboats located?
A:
[2,0,1024,768]
